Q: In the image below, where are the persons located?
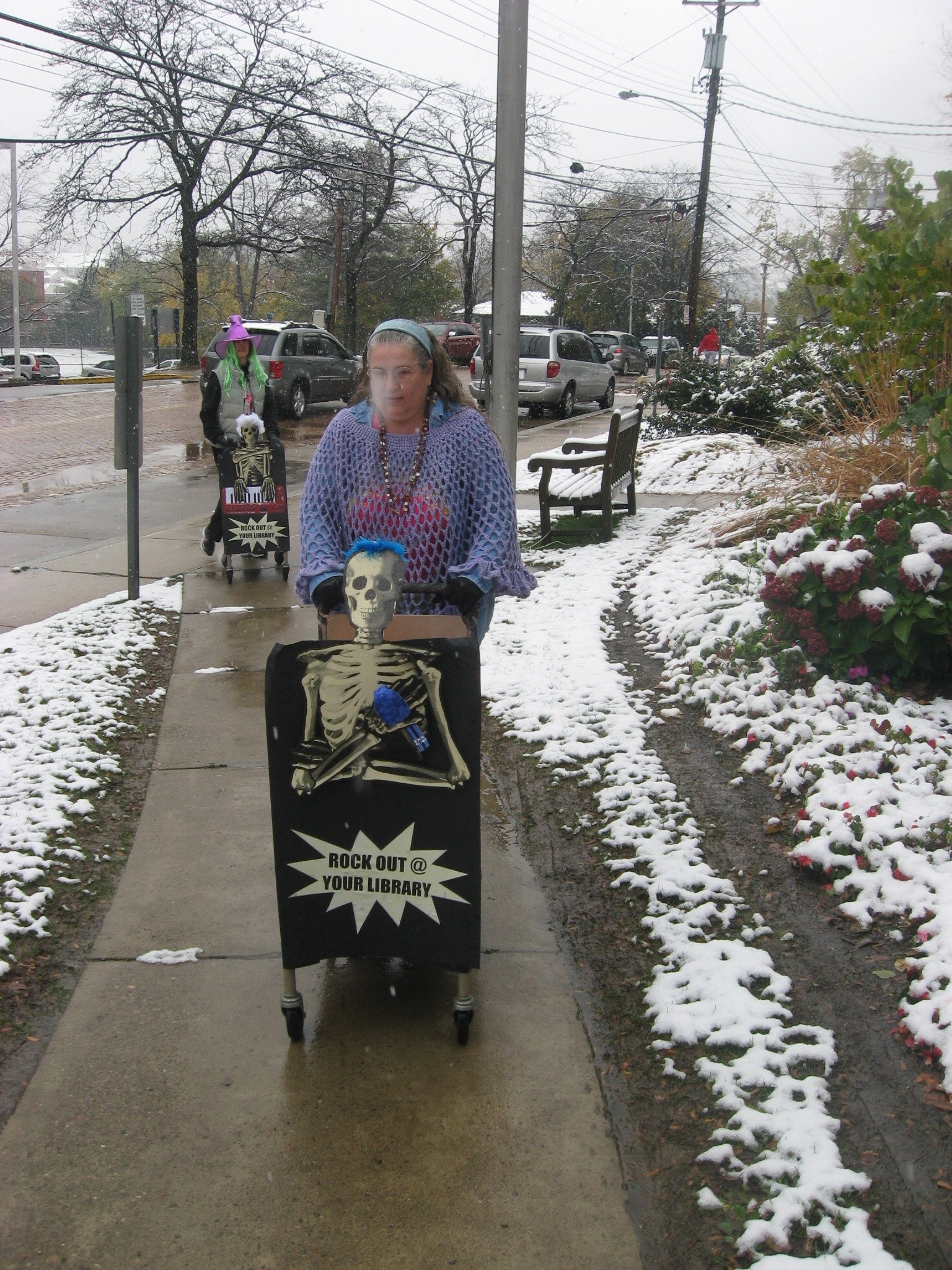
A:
[695,327,721,369]
[293,318,538,647]
[200,329,280,558]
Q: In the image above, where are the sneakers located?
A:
[241,552,267,558]
[200,526,216,556]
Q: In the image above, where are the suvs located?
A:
[469,321,617,419]
[589,330,650,375]
[419,321,478,367]
[641,335,682,368]
[0,353,60,381]
[200,316,363,420]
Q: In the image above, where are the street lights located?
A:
[615,2,732,348]
[151,305,161,365]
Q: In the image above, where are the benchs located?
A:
[527,399,645,547]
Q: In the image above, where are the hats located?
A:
[214,314,263,360]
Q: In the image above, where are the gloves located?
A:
[312,575,345,616]
[268,434,281,443]
[219,438,233,447]
[433,576,484,614]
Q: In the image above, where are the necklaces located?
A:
[373,403,432,516]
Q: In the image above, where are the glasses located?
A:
[367,361,421,382]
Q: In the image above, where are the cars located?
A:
[144,359,179,374]
[81,358,116,379]
[697,344,744,370]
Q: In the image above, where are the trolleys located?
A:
[214,387,290,581]
[262,538,485,1044]
